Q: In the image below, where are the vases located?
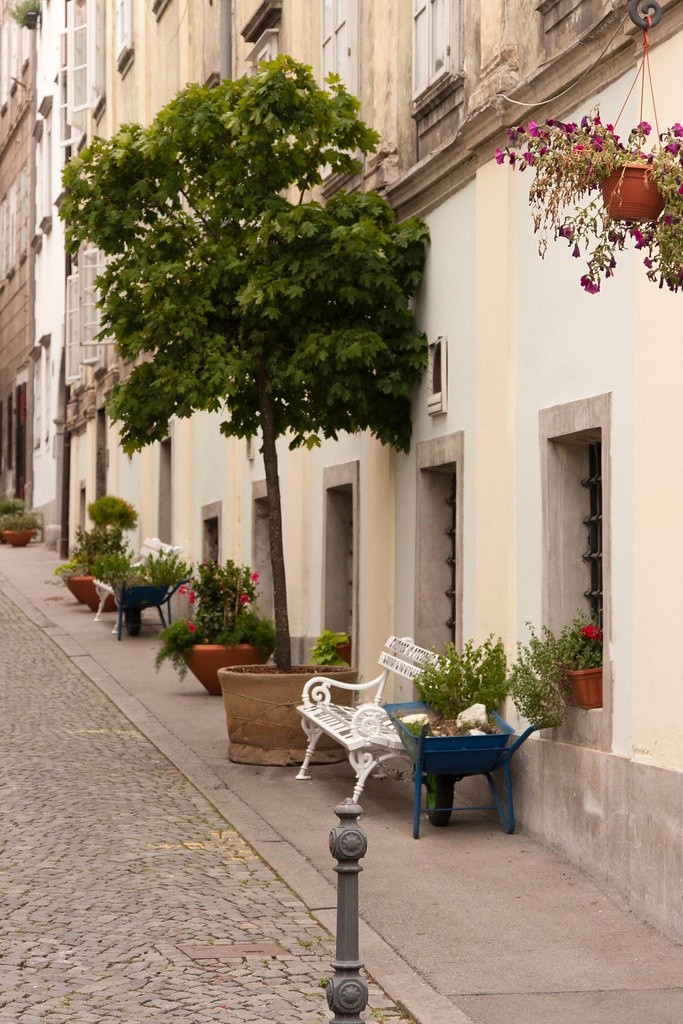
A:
[179,644,275,696]
[565,669,603,710]
[599,163,665,222]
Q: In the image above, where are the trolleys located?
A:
[383,702,558,839]
[117,579,191,641]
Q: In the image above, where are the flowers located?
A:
[153,561,279,683]
[508,608,603,728]
[496,101,683,297]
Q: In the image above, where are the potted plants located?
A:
[55,494,138,612]
[10,0,40,31]
[0,498,44,547]
[54,53,432,766]
[310,631,351,667]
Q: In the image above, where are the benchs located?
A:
[295,636,485,821]
[92,538,181,634]
